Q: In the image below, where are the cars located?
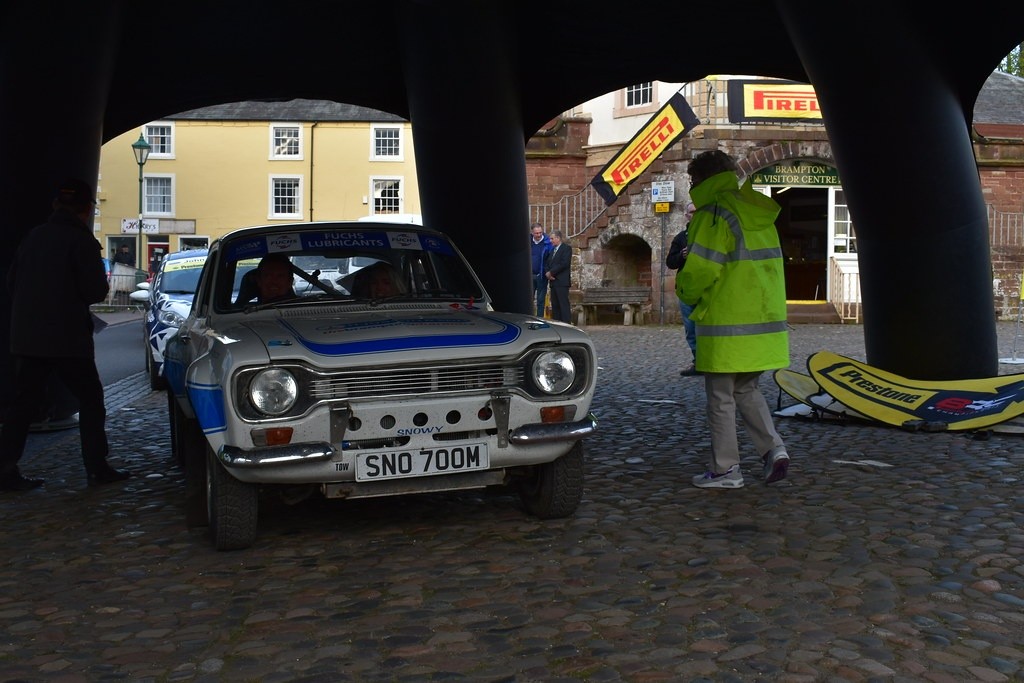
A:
[128,223,597,553]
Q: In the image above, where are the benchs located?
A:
[571,286,653,327]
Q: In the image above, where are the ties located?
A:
[552,249,556,259]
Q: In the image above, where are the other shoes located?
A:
[4,475,45,490]
[680,366,705,376]
[90,468,128,485]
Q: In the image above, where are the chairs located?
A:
[236,268,296,304]
[349,268,375,298]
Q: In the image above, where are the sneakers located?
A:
[764,446,791,483]
[692,464,744,488]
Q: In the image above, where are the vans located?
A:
[347,213,422,277]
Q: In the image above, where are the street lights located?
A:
[131,132,151,311]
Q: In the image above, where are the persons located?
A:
[530,222,572,325]
[255,255,303,306]
[675,151,791,489]
[111,244,134,267]
[665,202,703,377]
[358,261,410,300]
[182,242,208,251]
[0,179,129,492]
